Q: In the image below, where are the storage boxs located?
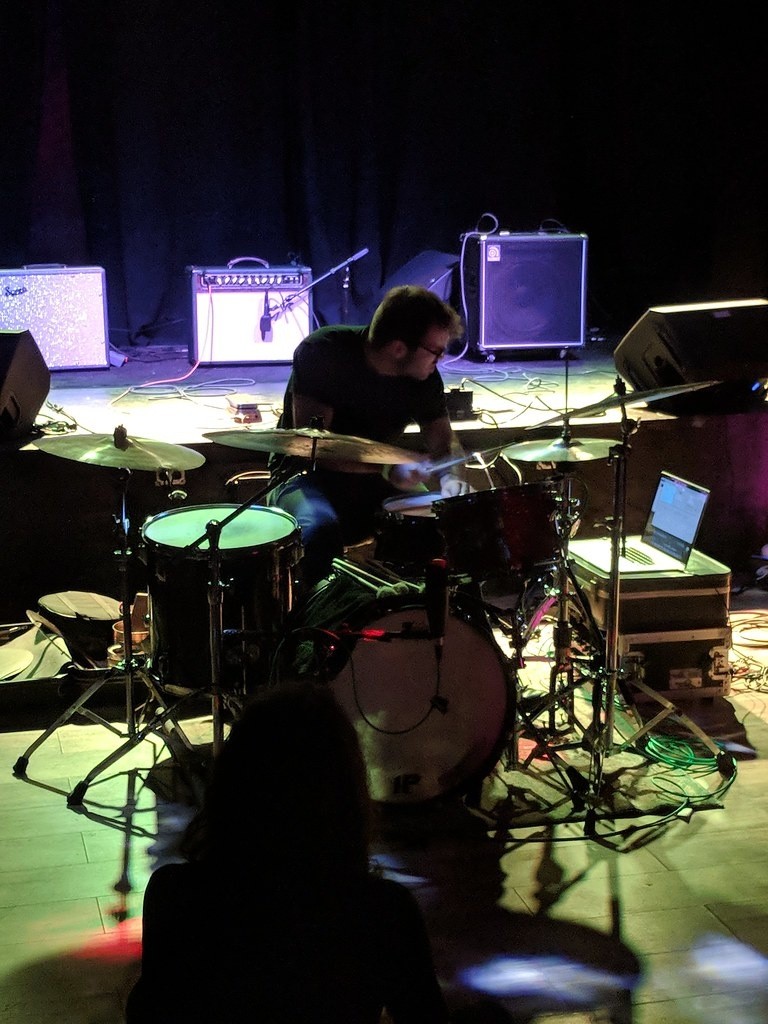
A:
[552,534,733,707]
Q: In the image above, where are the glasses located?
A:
[414,341,445,365]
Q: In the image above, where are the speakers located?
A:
[614,299,768,418]
[463,231,588,351]
[0,264,110,369]
[0,328,51,440]
[380,248,464,320]
[187,266,313,366]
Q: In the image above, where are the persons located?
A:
[267,284,470,599]
[135,684,451,1024]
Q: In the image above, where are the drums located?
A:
[431,481,571,591]
[139,500,304,695]
[380,487,452,585]
[304,574,519,819]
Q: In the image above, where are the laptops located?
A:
[566,470,710,574]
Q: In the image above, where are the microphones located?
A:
[422,562,449,661]
[260,291,271,331]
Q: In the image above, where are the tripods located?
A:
[12,444,318,807]
[509,400,738,828]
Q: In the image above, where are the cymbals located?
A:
[29,430,205,473]
[201,427,425,469]
[500,433,634,466]
[523,377,721,435]
